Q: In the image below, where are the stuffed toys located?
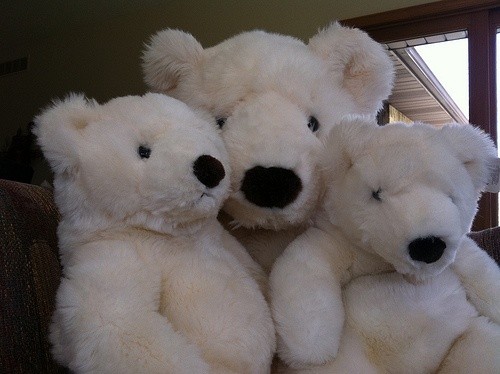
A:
[269,112,500,373]
[30,91,275,374]
[141,17,397,280]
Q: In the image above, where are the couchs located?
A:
[0,178,500,374]
